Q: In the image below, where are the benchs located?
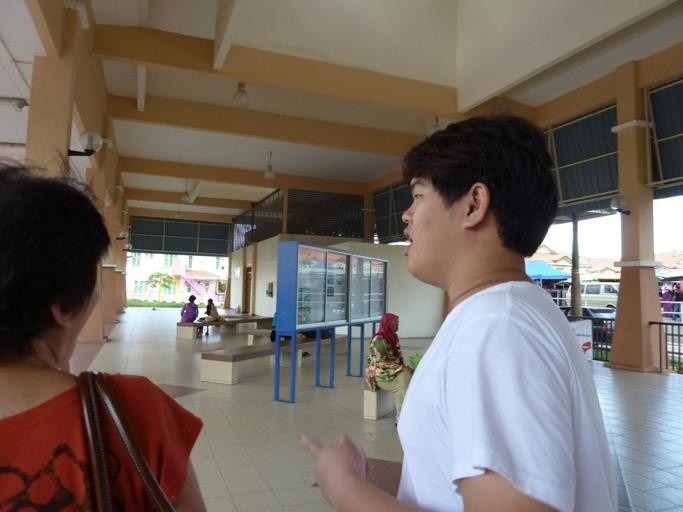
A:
[173,314,346,384]
[361,374,395,420]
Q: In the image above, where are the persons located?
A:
[1,156,206,512]
[198,299,219,335]
[658,282,683,320]
[301,115,619,511]
[365,313,413,425]
[181,296,198,324]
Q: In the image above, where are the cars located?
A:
[559,306,617,343]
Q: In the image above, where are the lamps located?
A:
[262,150,277,180]
[68,130,104,159]
[230,83,251,106]
[608,194,631,217]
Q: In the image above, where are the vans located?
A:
[566,282,620,310]
